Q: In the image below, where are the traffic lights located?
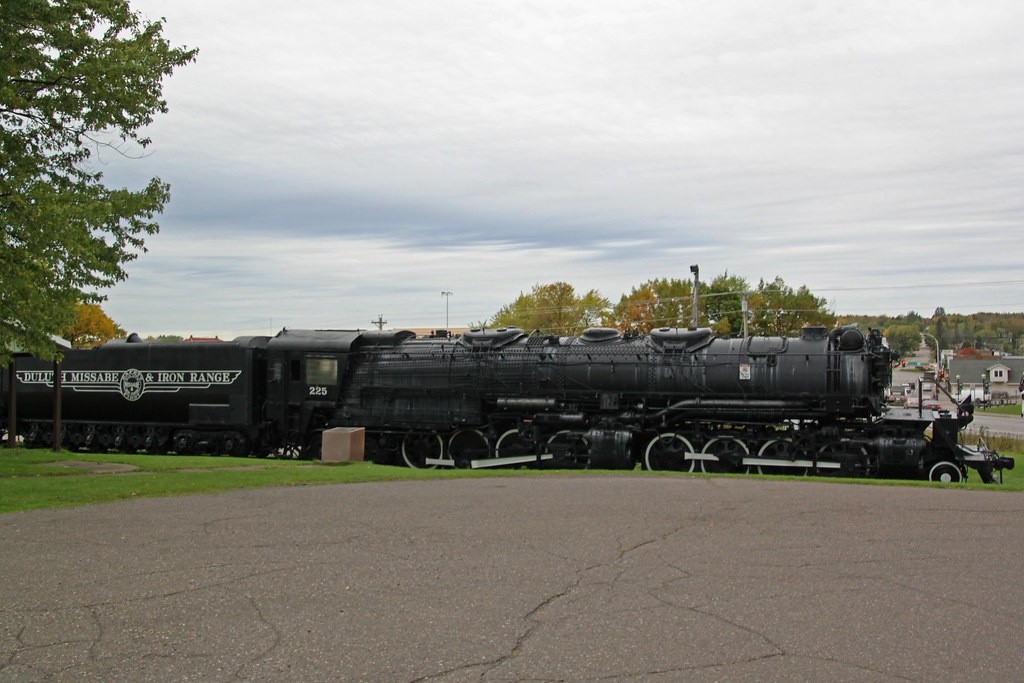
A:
[902,359,905,367]
[940,371,944,378]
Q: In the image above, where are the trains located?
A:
[0,322,1015,488]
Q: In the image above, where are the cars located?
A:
[885,369,942,410]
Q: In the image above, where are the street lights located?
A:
[981,372,986,411]
[441,290,454,331]
[688,263,700,328]
[919,332,940,370]
[956,374,960,404]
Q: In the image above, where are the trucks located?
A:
[951,385,991,405]
